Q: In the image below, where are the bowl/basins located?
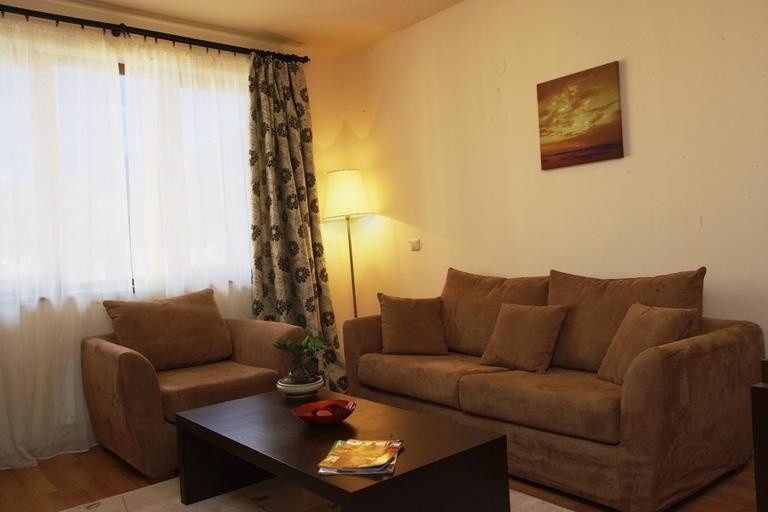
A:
[293,400,357,425]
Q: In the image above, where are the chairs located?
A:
[81,318,308,482]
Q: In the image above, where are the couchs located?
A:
[343,314,765,512]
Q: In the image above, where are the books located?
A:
[318,438,404,475]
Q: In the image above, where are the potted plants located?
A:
[273,336,337,401]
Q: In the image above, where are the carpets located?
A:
[56,473,575,512]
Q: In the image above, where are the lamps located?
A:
[320,168,378,318]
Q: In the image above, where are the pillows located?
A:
[440,267,549,358]
[102,288,234,371]
[376,292,449,355]
[479,300,570,375]
[546,265,707,373]
[595,301,699,386]
